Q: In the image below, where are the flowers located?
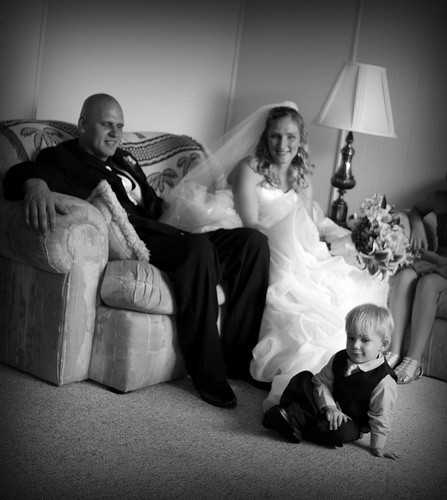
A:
[348,193,419,281]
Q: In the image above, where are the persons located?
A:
[387,189,447,384]
[4,91,272,409]
[261,303,403,462]
[226,105,368,419]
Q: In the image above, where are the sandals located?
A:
[383,351,423,384]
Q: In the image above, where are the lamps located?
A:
[313,62,397,224]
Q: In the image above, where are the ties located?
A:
[105,165,132,195]
[345,359,359,377]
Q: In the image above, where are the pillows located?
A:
[84,180,151,262]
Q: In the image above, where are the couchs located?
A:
[386,208,447,382]
[0,118,228,394]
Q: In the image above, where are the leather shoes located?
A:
[263,404,303,443]
[228,368,271,390]
[190,377,237,408]
